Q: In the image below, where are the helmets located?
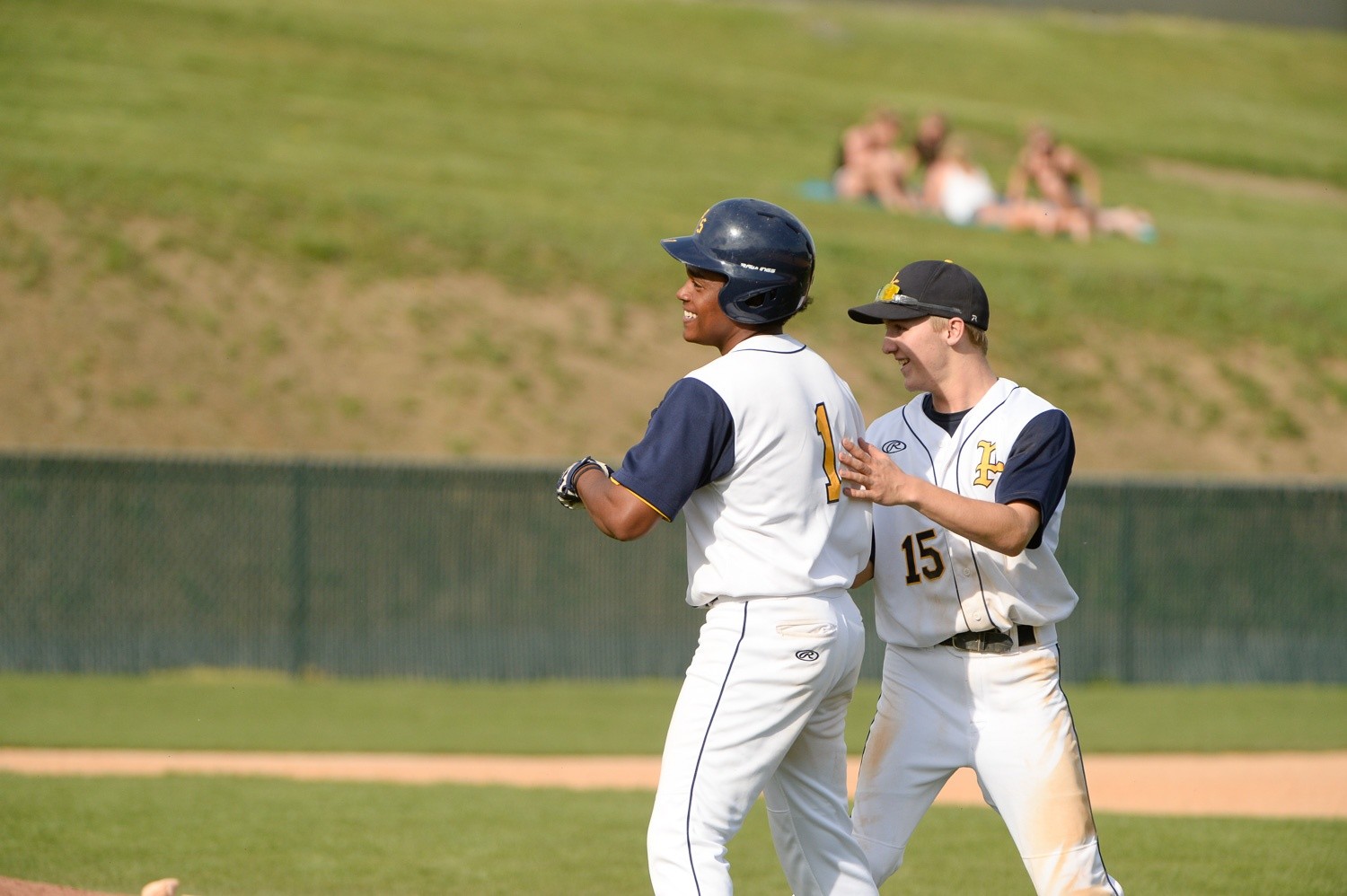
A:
[661,198,815,324]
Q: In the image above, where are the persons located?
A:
[552,201,877,896]
[830,102,1152,245]
[836,261,1119,896]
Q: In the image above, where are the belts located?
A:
[936,624,1036,652]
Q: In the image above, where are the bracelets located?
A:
[570,462,602,495]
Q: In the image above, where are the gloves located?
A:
[557,456,615,509]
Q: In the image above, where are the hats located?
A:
[848,259,989,332]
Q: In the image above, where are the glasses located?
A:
[874,282,961,314]
